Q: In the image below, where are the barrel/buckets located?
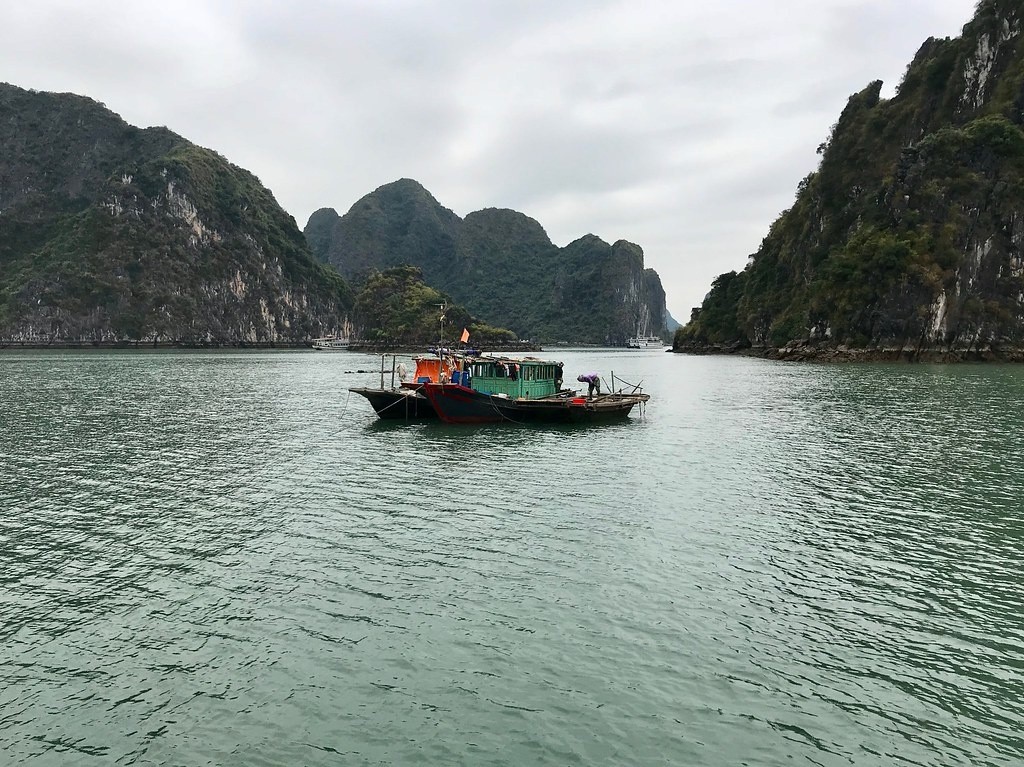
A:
[572,398,586,403]
[452,370,468,387]
[418,376,430,383]
[498,392,507,399]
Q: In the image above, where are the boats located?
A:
[626,336,665,351]
[344,346,484,420]
[310,334,350,350]
[398,352,650,426]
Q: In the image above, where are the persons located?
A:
[577,373,600,400]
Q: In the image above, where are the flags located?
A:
[461,327,470,344]
